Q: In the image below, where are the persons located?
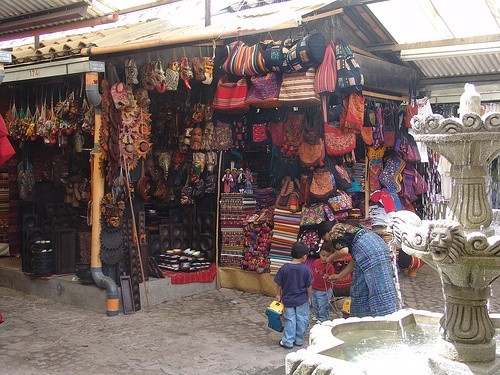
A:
[230,167,239,193]
[222,167,233,193]
[309,241,335,324]
[244,168,254,194]
[237,167,247,193]
[274,242,315,349]
[316,220,398,319]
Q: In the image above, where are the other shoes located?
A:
[278,339,303,350]
[311,316,323,325]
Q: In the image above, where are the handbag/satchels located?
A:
[211,24,434,273]
